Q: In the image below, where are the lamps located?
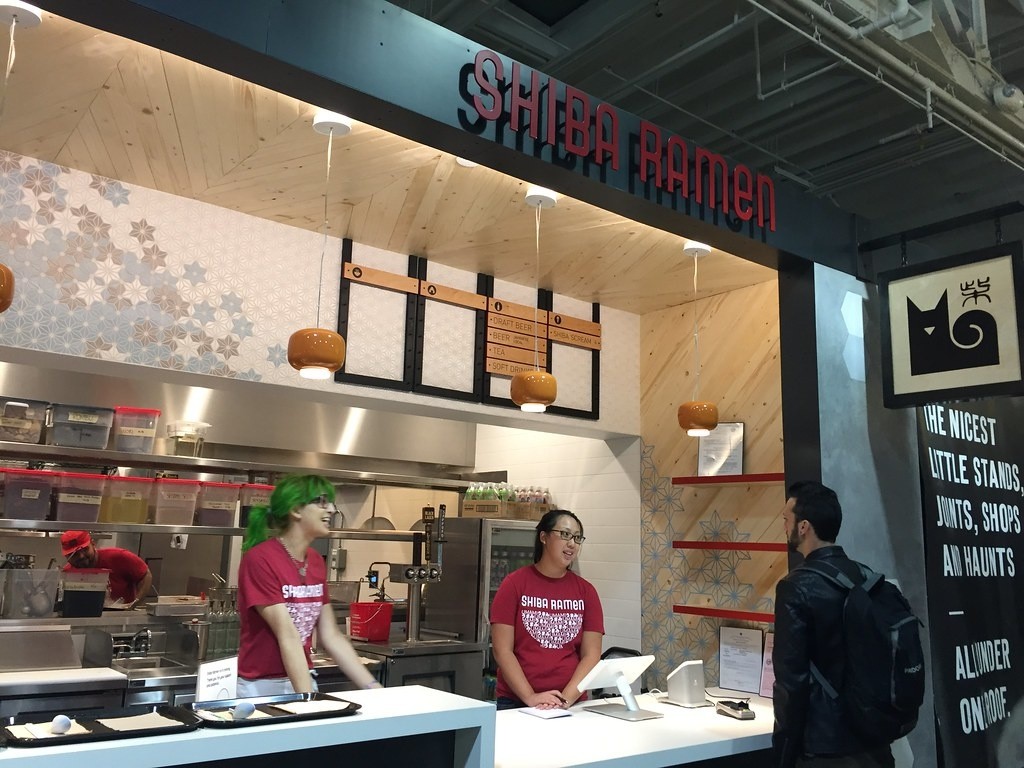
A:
[285,112,346,382]
[676,238,720,438]
[0,0,41,315]
[510,187,556,413]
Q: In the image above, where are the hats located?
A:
[60,530,90,555]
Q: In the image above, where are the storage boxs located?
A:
[348,601,393,641]
[0,468,66,522]
[40,399,116,450]
[148,477,202,525]
[193,480,241,527]
[530,502,557,521]
[0,395,50,444]
[98,475,156,525]
[240,482,277,529]
[517,501,531,520]
[0,569,113,618]
[48,472,109,522]
[462,500,519,519]
[114,406,161,455]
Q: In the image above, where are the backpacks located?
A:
[789,561,925,740]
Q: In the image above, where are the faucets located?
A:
[114,627,153,659]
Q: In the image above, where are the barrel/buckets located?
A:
[350,602,393,642]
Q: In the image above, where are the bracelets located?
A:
[365,680,379,690]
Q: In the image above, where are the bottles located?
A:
[205,600,242,659]
[463,482,552,506]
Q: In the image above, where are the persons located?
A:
[235,474,384,697]
[489,508,605,710]
[772,480,897,768]
[61,531,159,609]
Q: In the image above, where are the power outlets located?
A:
[368,571,379,588]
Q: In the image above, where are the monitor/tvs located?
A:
[577,655,656,692]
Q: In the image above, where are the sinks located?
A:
[111,656,188,672]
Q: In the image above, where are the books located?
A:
[518,702,572,718]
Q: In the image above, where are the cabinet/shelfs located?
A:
[672,473,787,623]
[1,440,500,541]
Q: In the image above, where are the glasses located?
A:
[547,530,585,544]
[310,494,329,508]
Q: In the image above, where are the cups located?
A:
[181,617,212,661]
